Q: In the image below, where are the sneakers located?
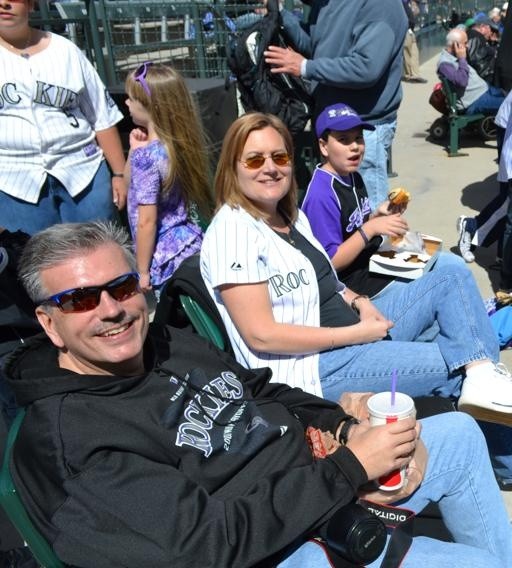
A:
[401,76,428,83]
[457,215,477,262]
[457,363,512,428]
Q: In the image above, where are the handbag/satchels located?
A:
[224,0,315,147]
[305,391,427,504]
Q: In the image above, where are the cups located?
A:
[367,392,416,493]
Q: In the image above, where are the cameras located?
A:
[320,499,388,566]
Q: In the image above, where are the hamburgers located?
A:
[388,187,410,207]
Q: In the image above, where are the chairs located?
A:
[176,283,233,358]
[3,398,66,567]
[437,71,490,158]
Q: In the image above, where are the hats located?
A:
[315,102,376,138]
[472,15,499,32]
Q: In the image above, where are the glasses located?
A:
[34,272,140,313]
[236,152,293,169]
[133,61,154,98]
